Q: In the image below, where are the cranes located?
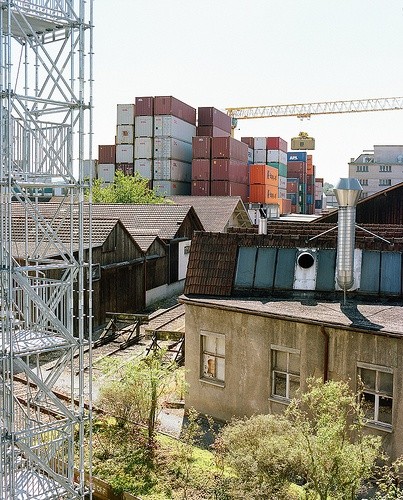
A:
[223,95,401,151]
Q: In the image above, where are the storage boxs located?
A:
[84,97,324,216]
